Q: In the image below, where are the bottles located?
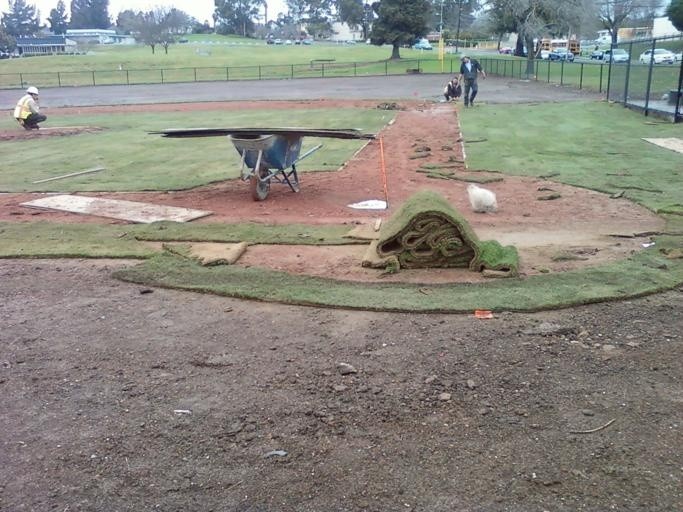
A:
[460,54,470,61]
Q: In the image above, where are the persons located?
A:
[455,54,487,108]
[440,77,462,103]
[13,87,45,130]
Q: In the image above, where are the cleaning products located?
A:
[229,132,323,201]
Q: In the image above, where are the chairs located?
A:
[464,102,473,108]
[24,123,39,130]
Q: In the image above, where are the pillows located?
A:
[26,86,39,95]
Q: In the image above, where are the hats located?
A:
[589,48,629,64]
[639,48,683,64]
[267,38,311,44]
[346,40,356,44]
[499,47,574,62]
[89,38,115,43]
[168,36,188,43]
[415,38,432,50]
